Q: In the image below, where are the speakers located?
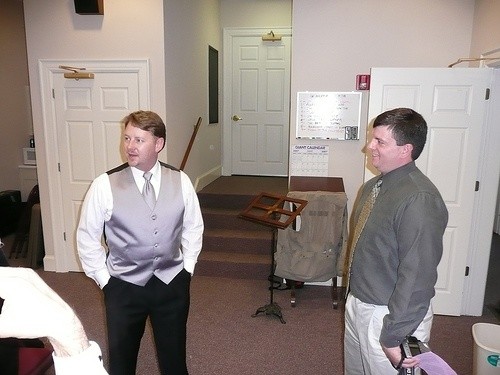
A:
[74,0,104,15]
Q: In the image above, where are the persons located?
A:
[343,107,449,375]
[75,110,205,375]
[0,266,109,375]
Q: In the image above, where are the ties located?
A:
[345,180,383,299]
[142,172,156,212]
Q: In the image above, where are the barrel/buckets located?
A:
[472,323,500,375]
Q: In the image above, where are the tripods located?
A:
[238,191,308,324]
